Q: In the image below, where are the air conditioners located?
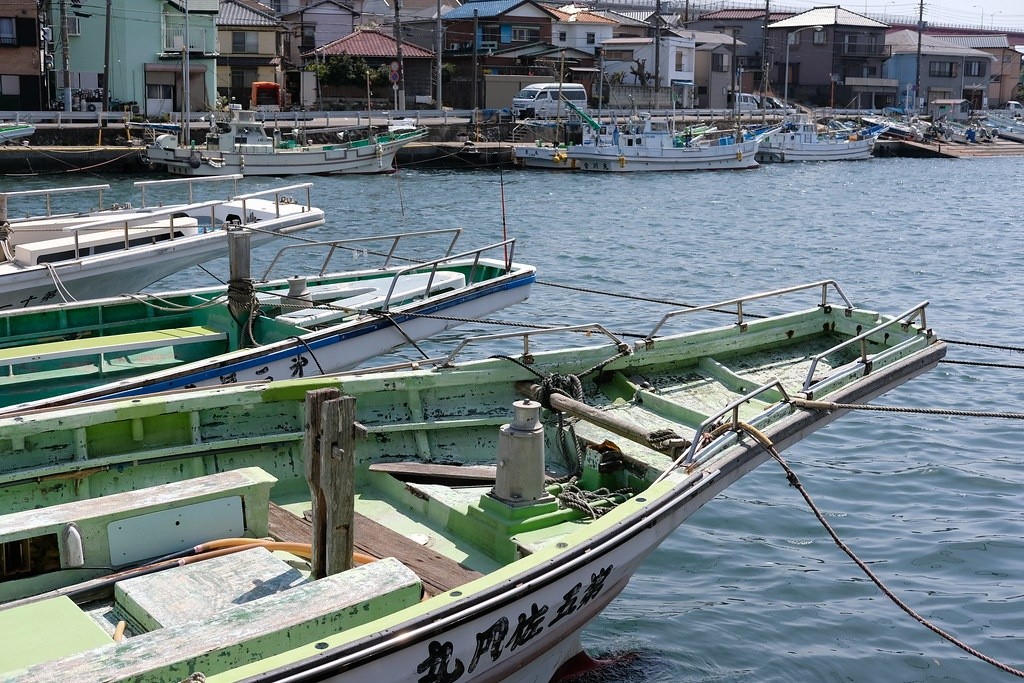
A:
[87,102,103,112]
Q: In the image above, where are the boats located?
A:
[1,277,934,678]
[0,172,325,311]
[513,50,1024,177]
[139,1,429,178]
[0,227,542,413]
[0,125,36,144]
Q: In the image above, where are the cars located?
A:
[752,94,772,109]
[763,96,791,109]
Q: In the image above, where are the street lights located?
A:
[884,1,895,22]
[990,11,1003,27]
[782,25,824,121]
[973,5,984,29]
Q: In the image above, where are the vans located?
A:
[733,92,758,109]
[511,82,587,119]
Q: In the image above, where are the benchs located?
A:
[0,325,229,370]
[0,466,278,605]
[14,215,199,265]
[0,557,422,683]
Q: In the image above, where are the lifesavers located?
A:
[985,130,991,139]
